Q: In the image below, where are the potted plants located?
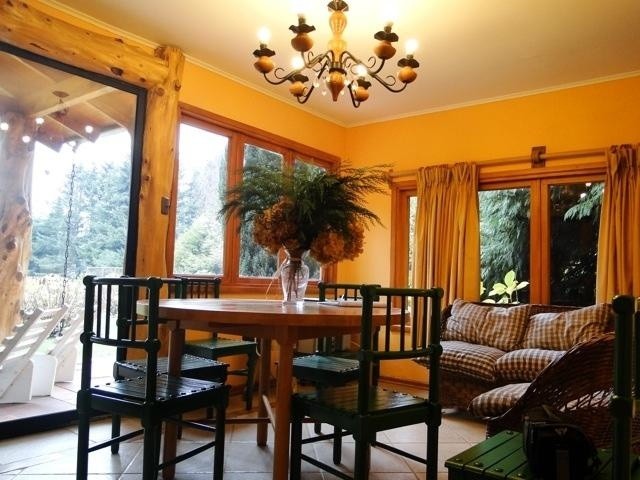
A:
[219,156,392,301]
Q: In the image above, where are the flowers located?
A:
[277,256,310,303]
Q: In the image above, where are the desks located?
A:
[130,297,413,479]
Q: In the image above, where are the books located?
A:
[316,299,394,308]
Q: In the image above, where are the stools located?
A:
[31,353,58,397]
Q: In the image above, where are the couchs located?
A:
[412,299,618,410]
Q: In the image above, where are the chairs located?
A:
[290,282,443,479]
[33,306,96,384]
[73,274,230,480]
[449,296,636,480]
[167,274,257,421]
[182,276,256,412]
[1,302,68,405]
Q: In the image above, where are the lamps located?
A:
[251,0,419,110]
